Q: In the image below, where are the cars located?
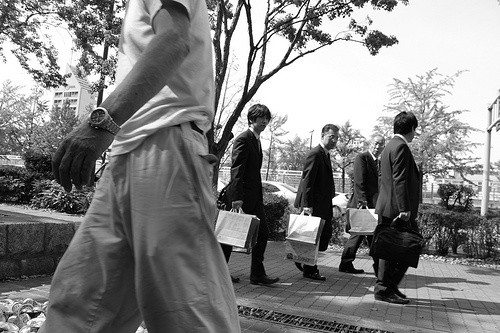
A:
[332,192,352,218]
[262,181,298,234]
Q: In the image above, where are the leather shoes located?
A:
[303,270,325,281]
[375,289,410,304]
[230,276,240,283]
[250,272,280,285]
[339,262,364,274]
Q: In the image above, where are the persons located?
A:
[370,111,421,304]
[218,104,281,285]
[294,124,339,281]
[36,0,244,332]
[338,136,386,274]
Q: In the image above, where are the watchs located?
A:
[87,107,120,136]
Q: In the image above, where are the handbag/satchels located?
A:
[215,207,261,248]
[284,210,322,244]
[345,205,379,235]
[283,211,325,266]
[369,215,424,269]
